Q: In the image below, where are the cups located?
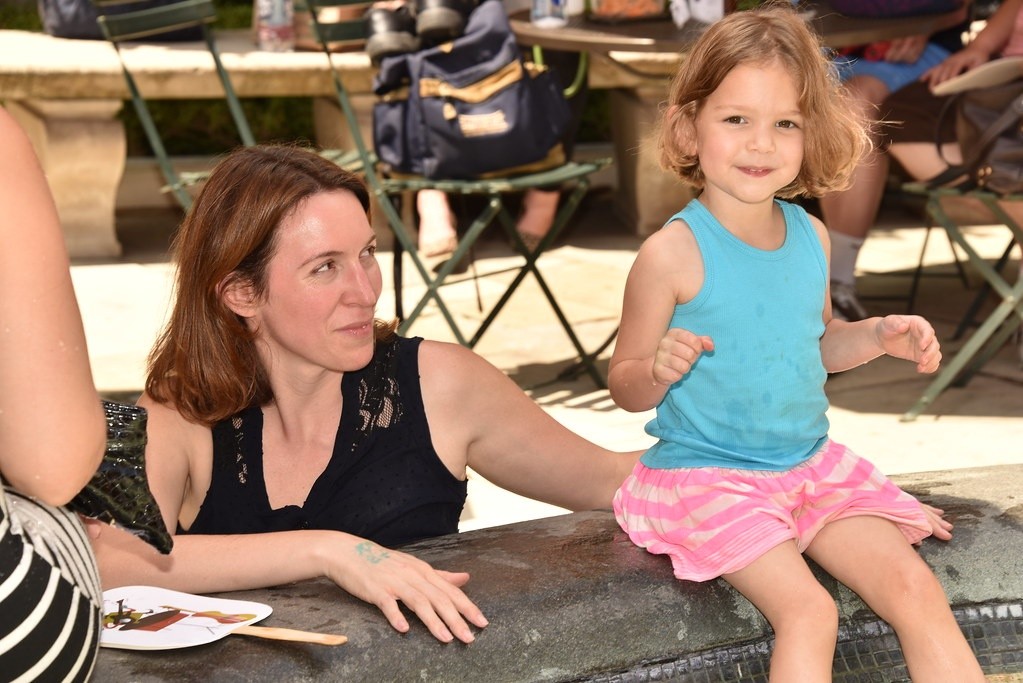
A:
[528,0,570,28]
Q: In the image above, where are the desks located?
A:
[585,40,694,237]
[0,28,420,268]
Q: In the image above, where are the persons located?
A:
[365,0,588,270]
[606,9,986,683]
[91,144,952,643]
[0,104,107,683]
[774,0,1023,321]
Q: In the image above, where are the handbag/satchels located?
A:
[372,1,568,186]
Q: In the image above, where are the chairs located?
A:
[89,0,1023,423]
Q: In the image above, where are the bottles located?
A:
[255,0,295,54]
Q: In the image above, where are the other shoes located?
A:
[364,3,417,60]
[408,0,464,46]
[509,226,540,252]
[419,229,458,271]
